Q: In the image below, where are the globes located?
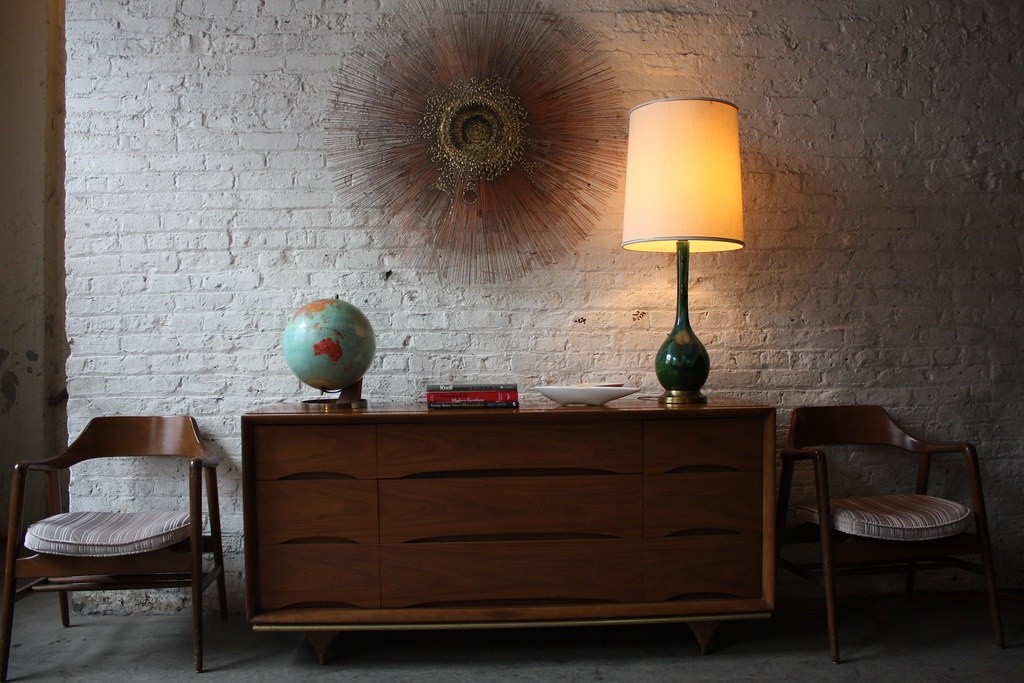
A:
[281,298,375,411]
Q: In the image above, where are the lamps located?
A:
[619,97,748,407]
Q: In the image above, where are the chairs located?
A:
[0,416,229,683]
[771,404,1008,666]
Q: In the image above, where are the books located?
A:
[426,382,519,409]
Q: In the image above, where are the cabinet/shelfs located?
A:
[241,398,776,665]
